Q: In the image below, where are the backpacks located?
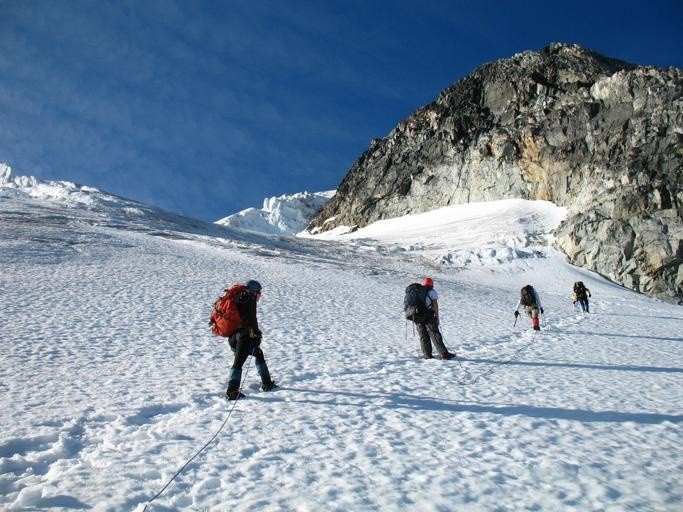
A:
[208,284,246,337]
[521,285,536,305]
[573,281,585,296]
[404,283,433,323]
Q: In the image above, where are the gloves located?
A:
[540,308,544,313]
[514,311,519,318]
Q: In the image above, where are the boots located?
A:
[534,326,540,330]
[261,376,278,391]
[424,353,434,359]
[439,352,455,360]
[226,380,246,399]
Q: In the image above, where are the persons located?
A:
[225,280,279,400]
[514,285,544,330]
[573,282,591,314]
[416,278,455,360]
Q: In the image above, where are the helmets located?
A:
[421,277,433,286]
[246,280,262,290]
